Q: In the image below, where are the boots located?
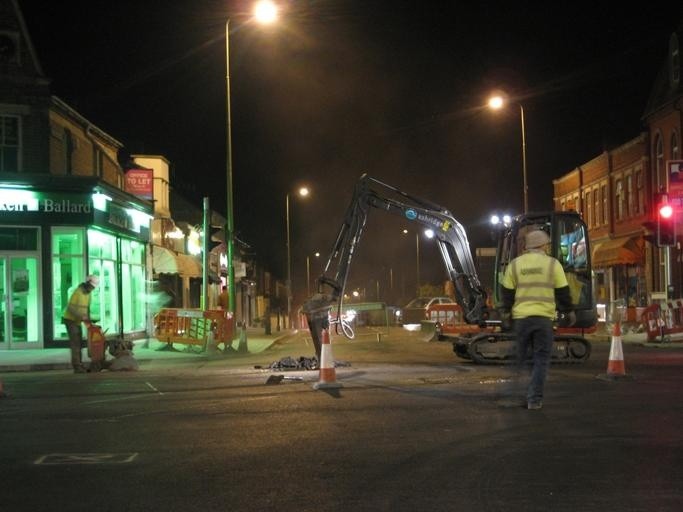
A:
[74,365,90,373]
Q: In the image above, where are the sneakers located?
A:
[528,403,544,409]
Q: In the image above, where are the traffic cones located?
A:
[312,328,342,392]
[236,320,251,353]
[203,322,221,360]
[597,322,640,382]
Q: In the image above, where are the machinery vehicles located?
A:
[299,170,598,366]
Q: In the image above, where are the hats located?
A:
[87,276,99,288]
[525,230,550,249]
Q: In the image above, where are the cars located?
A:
[395,296,456,326]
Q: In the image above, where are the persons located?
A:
[521,223,551,256]
[499,228,573,411]
[59,274,100,375]
[215,284,229,311]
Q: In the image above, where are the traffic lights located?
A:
[659,204,676,247]
[642,218,658,248]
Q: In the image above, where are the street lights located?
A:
[223,2,281,320]
[286,187,308,330]
[404,229,420,286]
[306,253,321,298]
[488,95,530,213]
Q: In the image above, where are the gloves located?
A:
[568,310,576,327]
[501,313,512,330]
